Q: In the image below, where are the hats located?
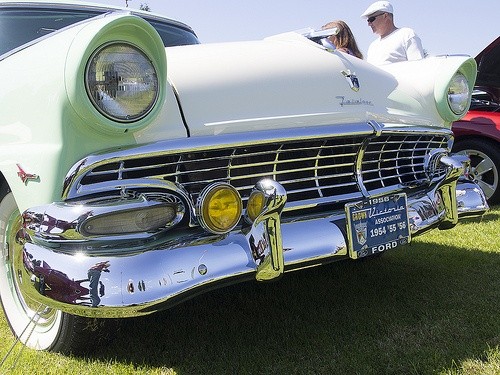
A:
[360,1,393,20]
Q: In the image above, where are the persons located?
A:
[318,19,364,60]
[359,1,426,62]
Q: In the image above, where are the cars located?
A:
[0,1,478,356]
[452,36,500,204]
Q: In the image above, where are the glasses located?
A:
[367,12,386,22]
